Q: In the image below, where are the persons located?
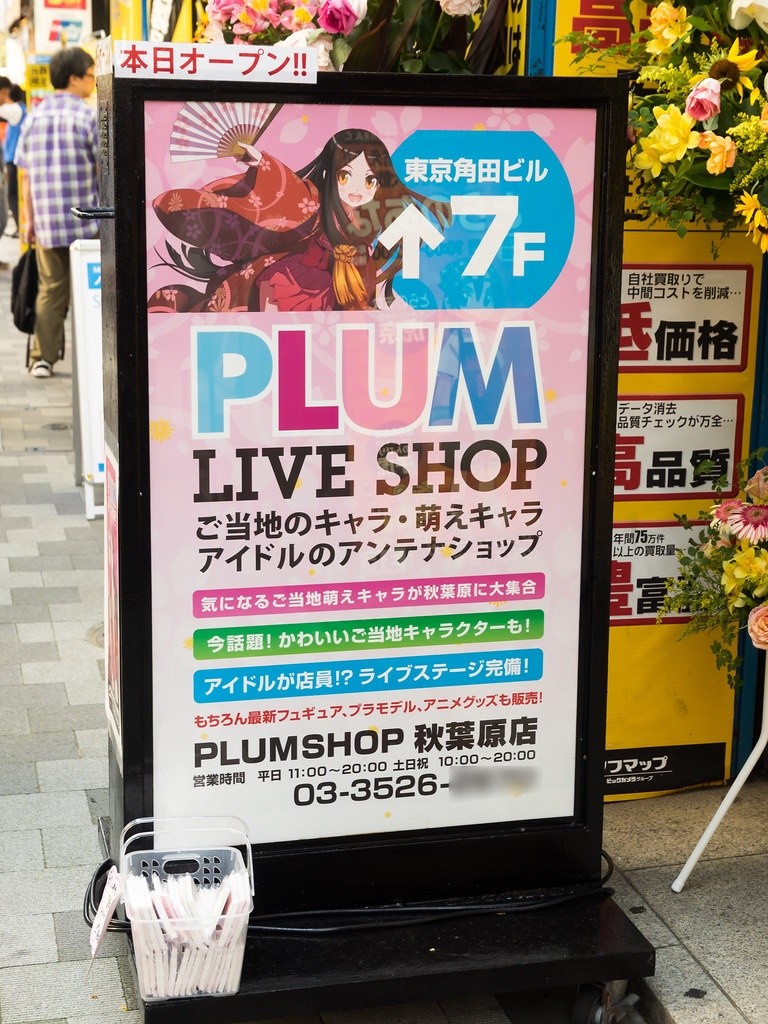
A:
[12,46,99,378]
[0,76,27,270]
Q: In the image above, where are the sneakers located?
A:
[31,359,51,377]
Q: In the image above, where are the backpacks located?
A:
[10,242,38,334]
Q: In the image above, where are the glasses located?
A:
[85,74,95,81]
